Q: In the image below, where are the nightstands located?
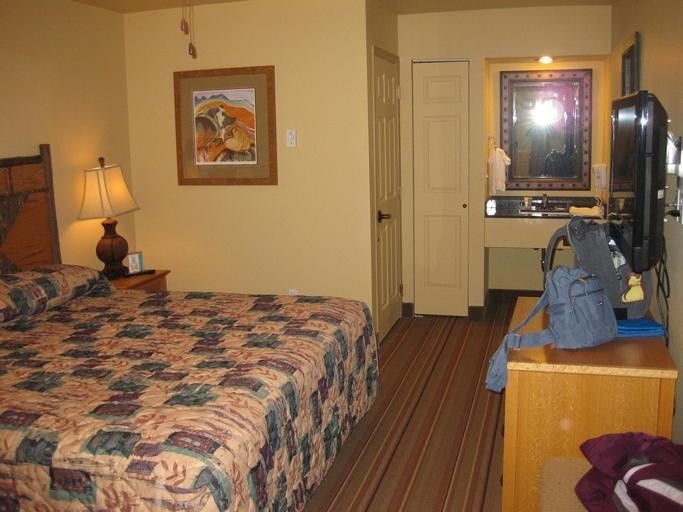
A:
[109,268,172,293]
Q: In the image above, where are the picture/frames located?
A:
[171,62,278,186]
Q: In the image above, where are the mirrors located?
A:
[496,66,593,191]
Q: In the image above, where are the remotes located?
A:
[123,270,156,277]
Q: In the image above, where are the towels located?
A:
[566,205,601,218]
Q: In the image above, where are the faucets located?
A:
[540,193,548,210]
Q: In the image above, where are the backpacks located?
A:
[543,214,654,321]
[541,264,619,350]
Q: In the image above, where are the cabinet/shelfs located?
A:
[492,294,678,512]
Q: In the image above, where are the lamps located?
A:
[77,155,138,279]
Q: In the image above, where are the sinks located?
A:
[519,200,572,213]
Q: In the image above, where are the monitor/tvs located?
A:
[608,90,669,275]
[564,112,574,157]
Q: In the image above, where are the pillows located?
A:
[1,262,110,321]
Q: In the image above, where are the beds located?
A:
[0,141,380,511]
